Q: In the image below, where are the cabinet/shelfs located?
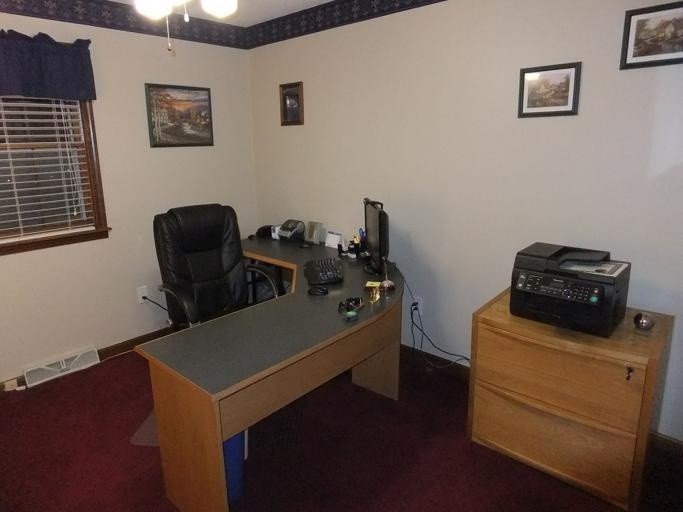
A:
[471,284,675,512]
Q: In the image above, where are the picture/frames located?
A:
[278,82,305,126]
[144,82,215,149]
[518,60,582,118]
[619,0,683,71]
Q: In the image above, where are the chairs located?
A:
[153,202,279,334]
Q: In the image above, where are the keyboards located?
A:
[303,255,343,285]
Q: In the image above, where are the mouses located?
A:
[308,285,328,295]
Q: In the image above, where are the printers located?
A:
[509,242,631,338]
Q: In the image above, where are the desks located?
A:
[133,231,405,512]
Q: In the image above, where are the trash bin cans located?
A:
[223,432,247,502]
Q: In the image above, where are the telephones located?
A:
[257,225,273,236]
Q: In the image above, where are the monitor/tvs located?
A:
[363,198,389,275]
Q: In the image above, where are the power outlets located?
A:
[137,286,150,305]
